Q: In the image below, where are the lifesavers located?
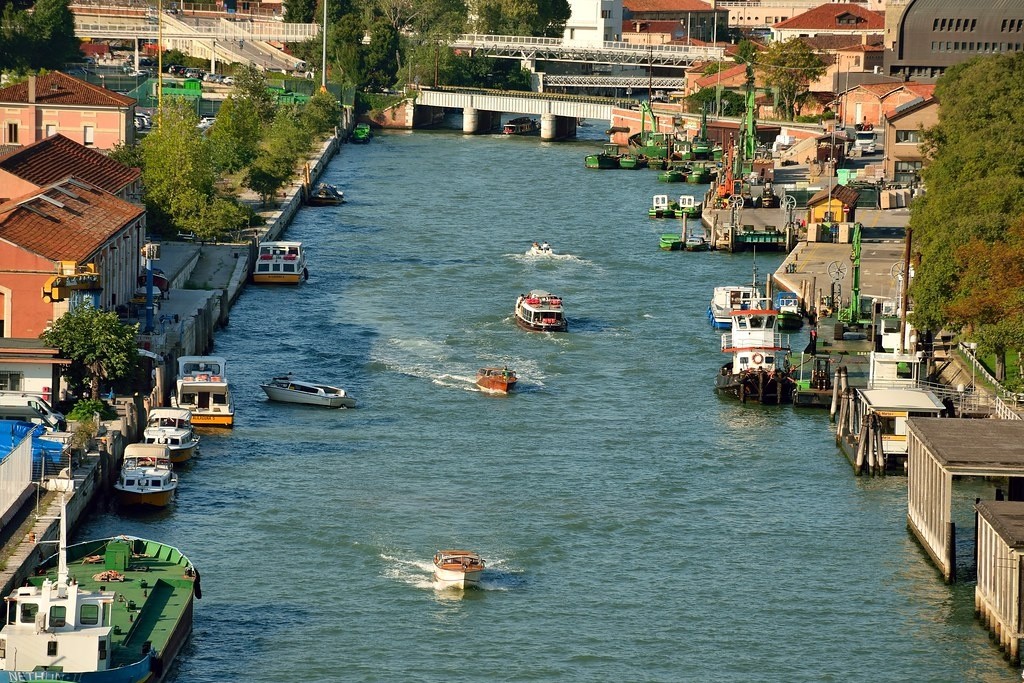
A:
[751,353,763,364]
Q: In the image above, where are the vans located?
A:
[0,393,68,433]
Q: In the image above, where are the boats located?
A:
[135,267,172,299]
[258,375,357,408]
[706,284,762,330]
[712,309,791,402]
[253,241,308,287]
[576,117,586,127]
[431,548,484,590]
[0,488,203,683]
[685,234,708,252]
[111,443,179,512]
[309,183,346,207]
[475,366,516,396]
[659,233,682,251]
[350,122,373,144]
[774,291,804,330]
[169,355,236,426]
[648,194,679,218]
[514,287,569,333]
[584,64,763,184]
[674,194,703,219]
[503,116,541,135]
[139,406,201,463]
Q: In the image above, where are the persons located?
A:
[533,242,541,250]
[518,293,525,303]
[542,241,550,248]
[501,365,508,370]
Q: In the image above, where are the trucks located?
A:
[854,130,877,154]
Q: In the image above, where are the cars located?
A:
[121,57,241,133]
